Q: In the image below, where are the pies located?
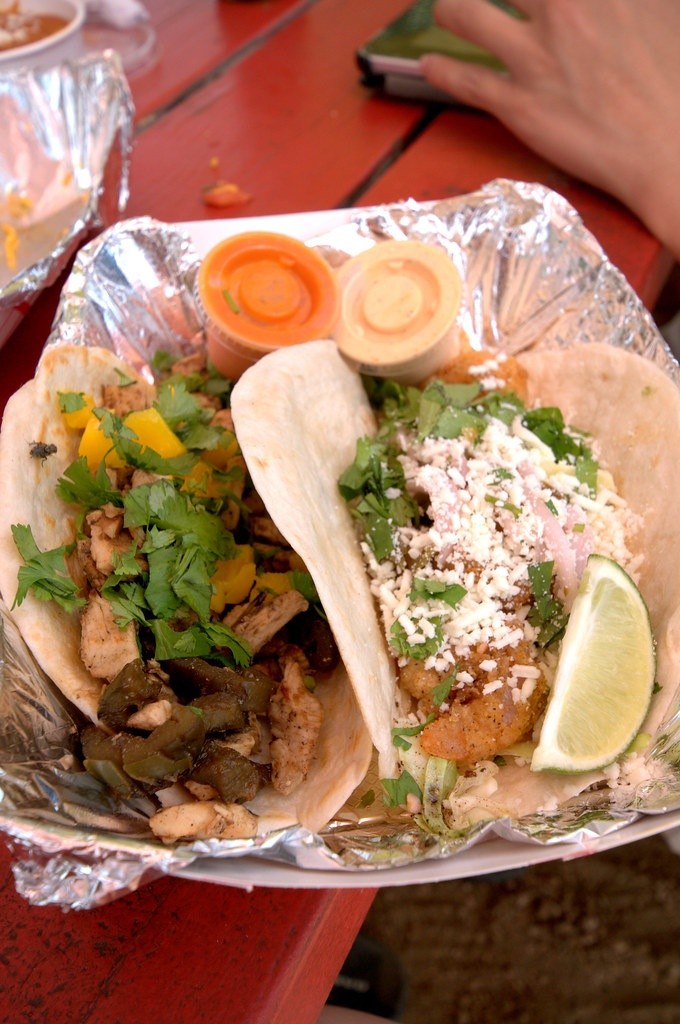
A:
[1,337,680,841]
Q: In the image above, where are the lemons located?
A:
[529,554,656,774]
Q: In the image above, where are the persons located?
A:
[420,0,680,254]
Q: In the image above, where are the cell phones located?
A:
[355,0,529,113]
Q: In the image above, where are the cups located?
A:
[1,0,86,65]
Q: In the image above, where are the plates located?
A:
[0,344,680,889]
